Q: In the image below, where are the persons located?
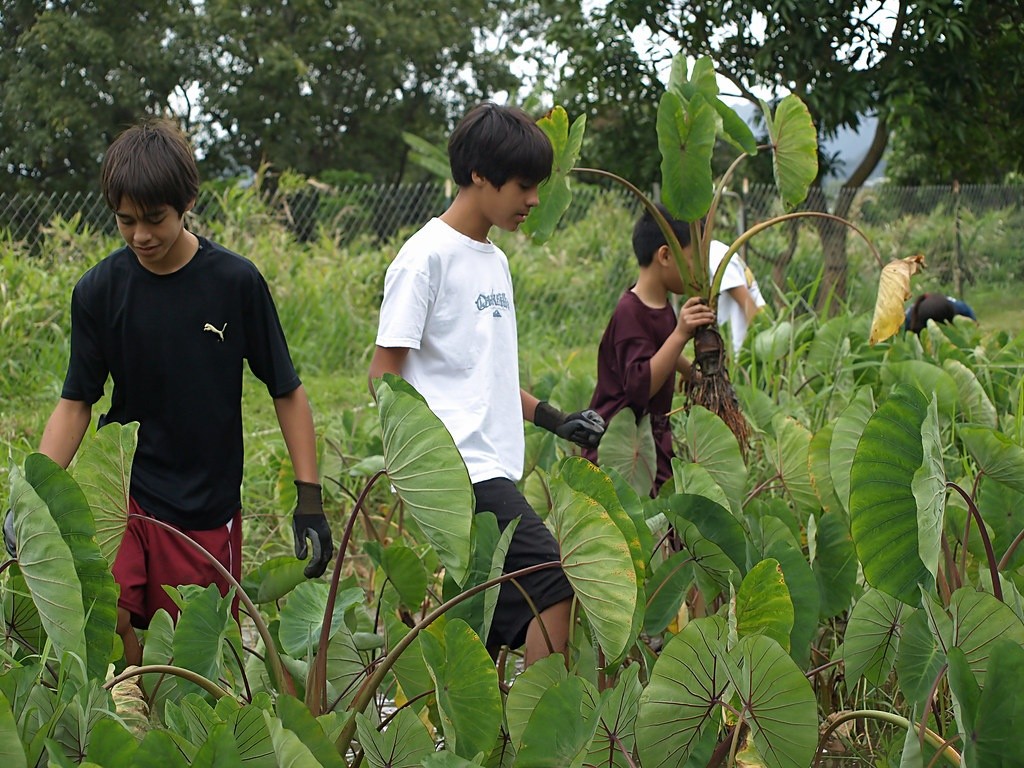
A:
[3,119,333,699]
[581,203,715,553]
[906,292,976,338]
[710,240,765,364]
[368,104,606,672]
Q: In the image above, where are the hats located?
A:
[903,294,975,337]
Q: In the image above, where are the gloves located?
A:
[2,510,17,558]
[532,401,606,449]
[290,480,334,578]
